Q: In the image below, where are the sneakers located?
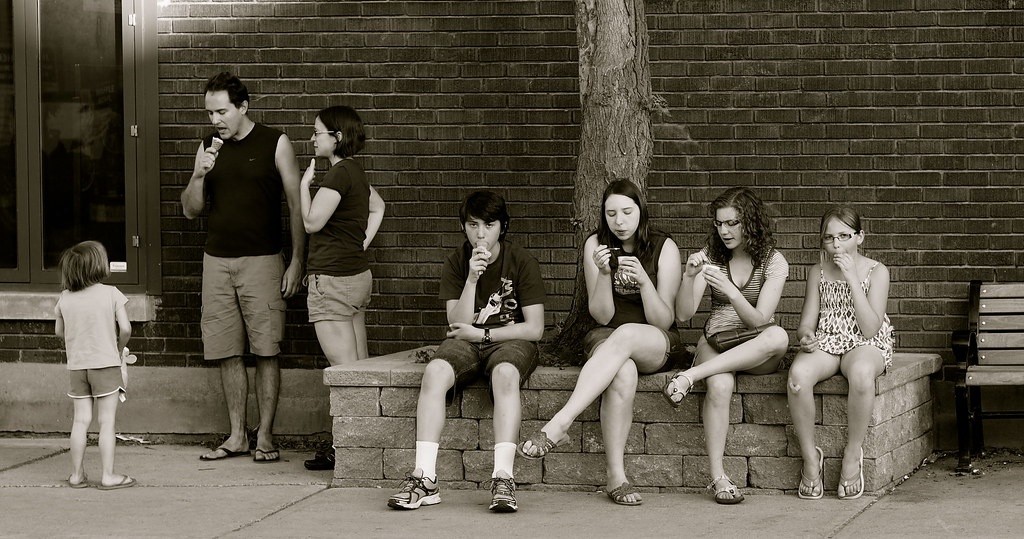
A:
[387,468,442,509]
[482,468,518,512]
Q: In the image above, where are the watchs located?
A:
[482,328,492,344]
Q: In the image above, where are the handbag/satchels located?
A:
[708,322,779,353]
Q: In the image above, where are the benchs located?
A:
[953,282,1024,468]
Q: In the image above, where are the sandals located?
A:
[662,369,694,408]
[707,475,744,504]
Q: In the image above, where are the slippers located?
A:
[606,482,643,506]
[199,447,251,462]
[254,449,280,463]
[98,473,137,490]
[68,471,88,488]
[798,446,824,500]
[516,429,570,459]
[837,446,864,499]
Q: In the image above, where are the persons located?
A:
[387,190,547,511]
[662,186,789,504]
[787,207,895,499]
[181,68,306,463]
[300,106,386,470]
[54,240,137,490]
[516,178,683,506]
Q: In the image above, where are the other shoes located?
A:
[304,452,336,471]
[315,445,335,457]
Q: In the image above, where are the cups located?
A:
[702,265,720,272]
[617,256,634,265]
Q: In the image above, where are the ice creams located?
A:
[476,241,488,274]
[211,137,223,155]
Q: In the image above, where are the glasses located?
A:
[821,231,858,244]
[712,219,741,228]
[312,129,334,137]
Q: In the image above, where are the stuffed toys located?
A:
[121,346,137,389]
